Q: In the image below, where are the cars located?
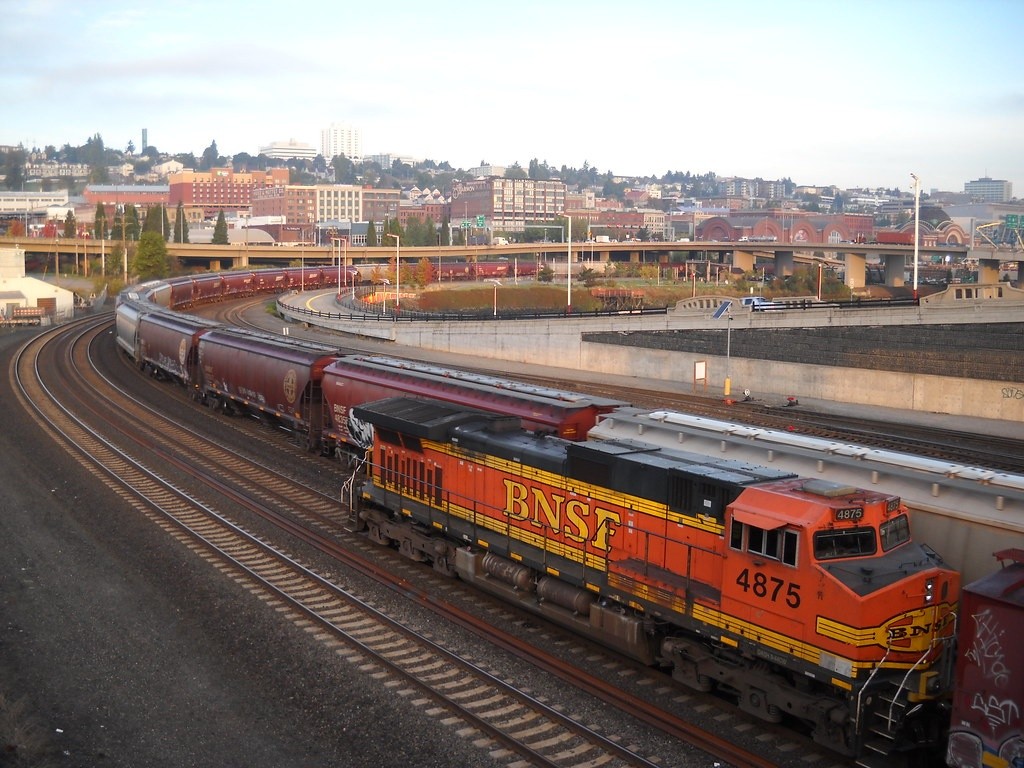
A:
[839,240,854,244]
[739,236,748,242]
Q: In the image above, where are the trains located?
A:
[340,398,1023,768]
[115,259,1023,611]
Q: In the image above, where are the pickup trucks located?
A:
[741,296,786,312]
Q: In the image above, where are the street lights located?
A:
[386,233,400,316]
[562,215,572,314]
[435,232,441,286]
[330,238,347,294]
